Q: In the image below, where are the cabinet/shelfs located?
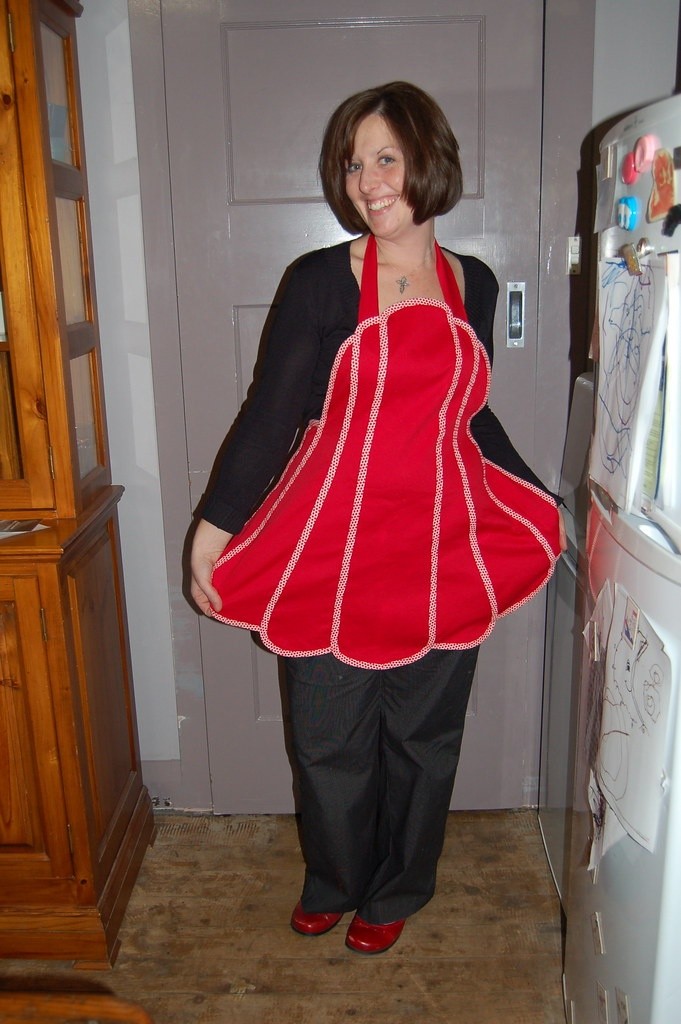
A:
[0,485,160,971]
[0,0,113,520]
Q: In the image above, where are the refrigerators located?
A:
[559,88,681,1024]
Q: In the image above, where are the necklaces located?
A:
[374,240,436,295]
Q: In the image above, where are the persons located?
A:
[187,82,569,953]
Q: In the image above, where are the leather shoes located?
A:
[290,897,344,936]
[345,911,406,955]
